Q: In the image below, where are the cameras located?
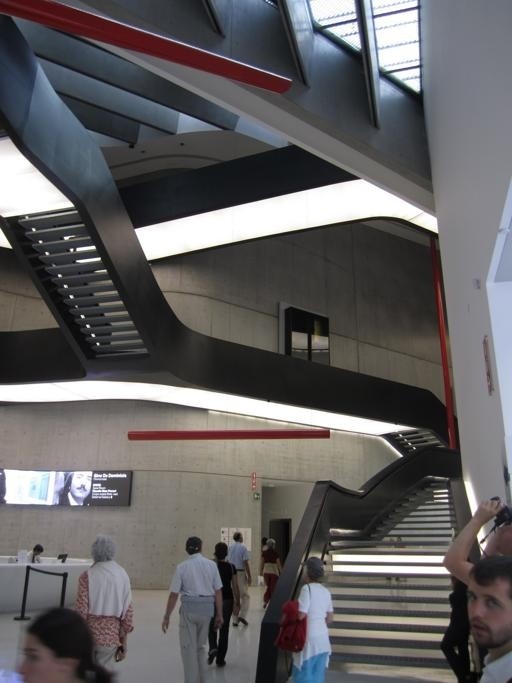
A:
[490,496,512,527]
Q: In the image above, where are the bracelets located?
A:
[164,613,169,619]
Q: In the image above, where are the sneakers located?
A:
[208,649,218,665]
[232,617,248,626]
[216,661,226,666]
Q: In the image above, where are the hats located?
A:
[307,556,324,577]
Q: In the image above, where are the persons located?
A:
[75,535,134,682]
[260,537,283,608]
[466,555,511,681]
[53,471,93,506]
[287,555,335,682]
[23,542,44,563]
[224,532,252,626]
[440,557,475,683]
[161,536,225,682]
[442,499,511,587]
[207,541,241,665]
[18,606,121,681]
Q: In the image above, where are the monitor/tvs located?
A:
[57,553,67,563]
